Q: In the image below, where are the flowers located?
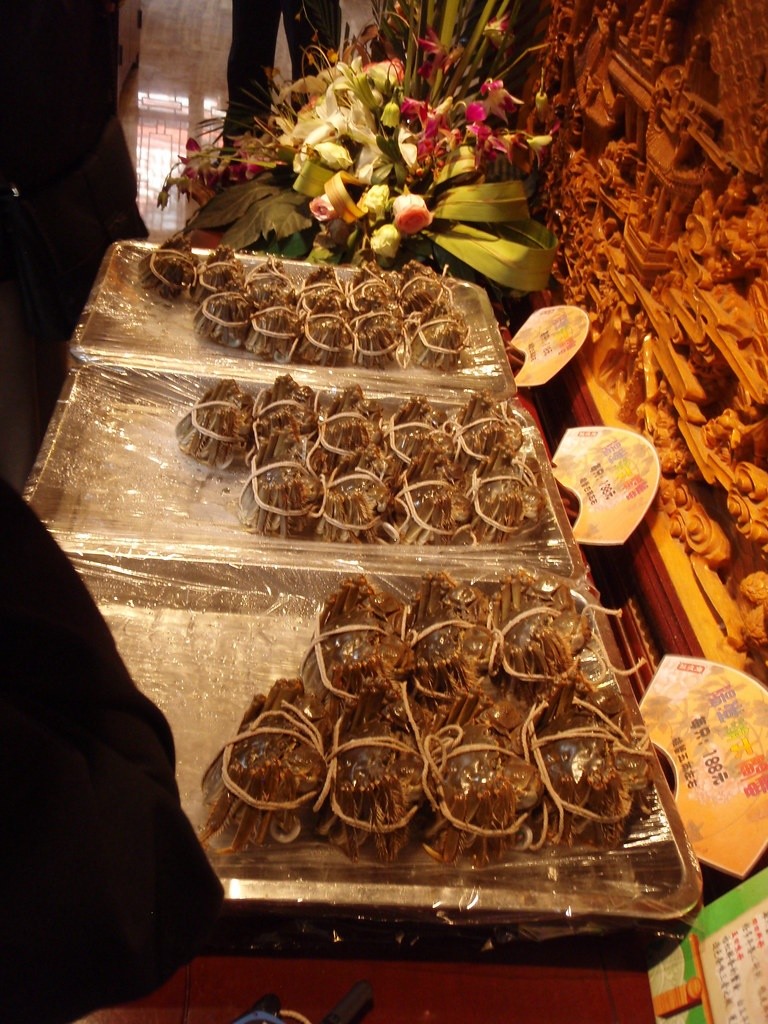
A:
[157,1,555,293]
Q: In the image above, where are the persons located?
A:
[0,0,119,282]
[224,0,343,149]
[0,478,221,1023]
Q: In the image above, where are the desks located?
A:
[84,223,660,1024]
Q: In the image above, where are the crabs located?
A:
[382,395,451,468]
[489,571,594,698]
[302,570,409,717]
[320,383,380,449]
[194,288,470,372]
[202,676,328,856]
[318,450,393,543]
[321,674,433,864]
[192,241,453,314]
[248,448,318,536]
[531,701,651,851]
[449,392,521,474]
[425,689,541,867]
[392,452,469,546]
[466,448,545,545]
[253,374,318,443]
[399,571,495,690]
[176,378,255,466]
[141,227,197,300]
